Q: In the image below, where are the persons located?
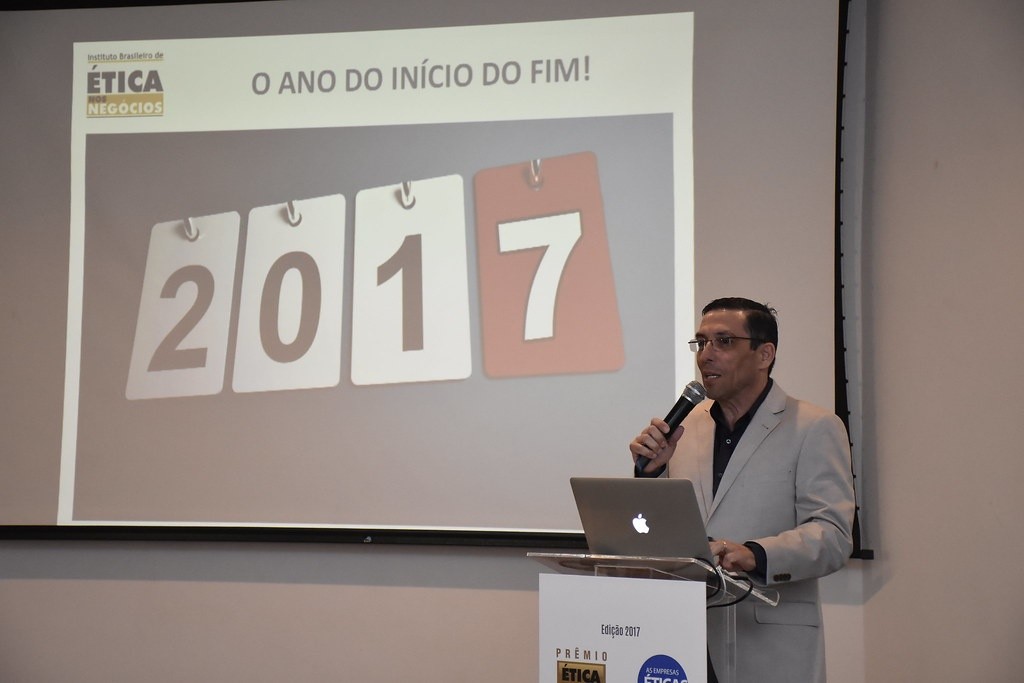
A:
[628,297,855,683]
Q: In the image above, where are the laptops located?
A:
[570,477,715,569]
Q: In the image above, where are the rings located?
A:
[723,541,728,552]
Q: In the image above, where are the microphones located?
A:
[636,380,707,472]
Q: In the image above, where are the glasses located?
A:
[688,335,751,351]
